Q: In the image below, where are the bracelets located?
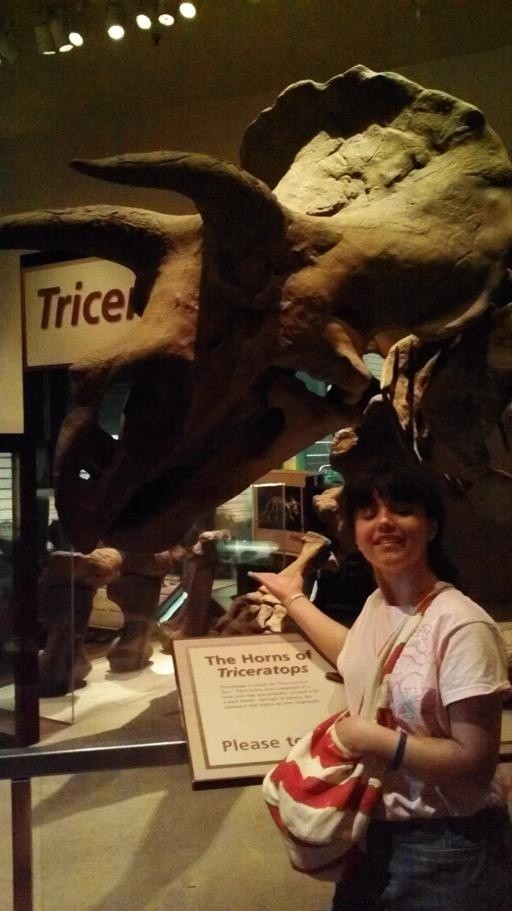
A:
[285,593,306,608]
[390,730,407,770]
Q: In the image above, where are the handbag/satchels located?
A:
[261,708,393,882]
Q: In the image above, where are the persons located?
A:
[247,463,512,911]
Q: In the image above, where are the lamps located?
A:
[2,1,200,66]
